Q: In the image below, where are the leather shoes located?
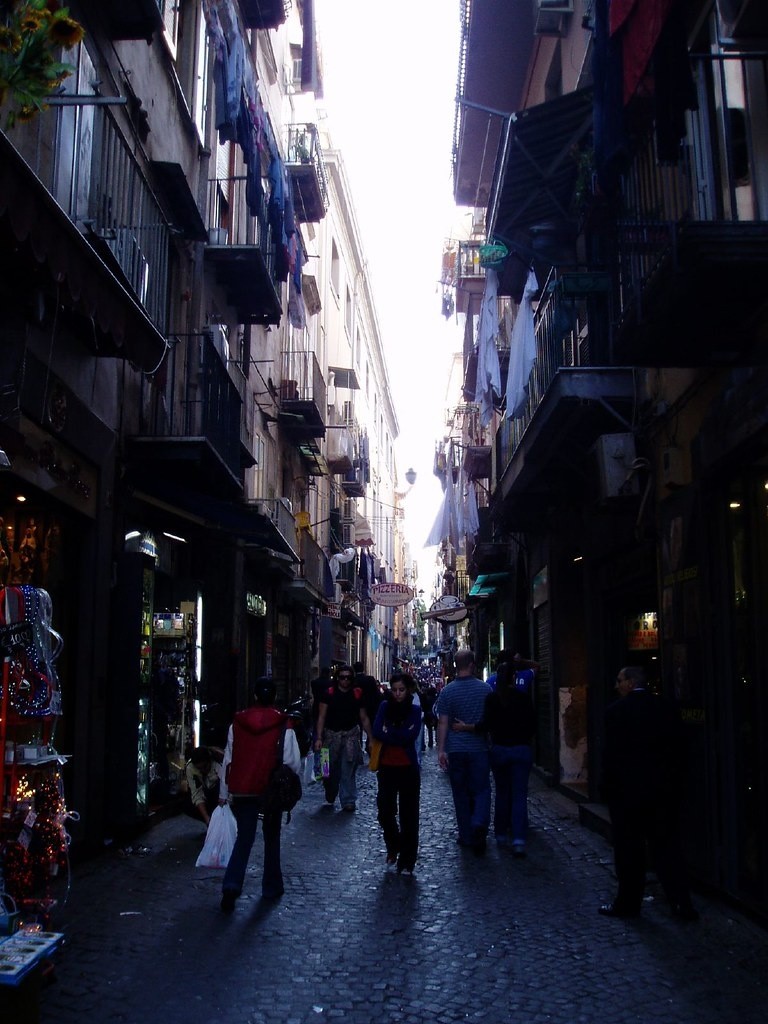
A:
[218,890,284,906]
[598,903,696,920]
[328,797,355,812]
[421,742,439,750]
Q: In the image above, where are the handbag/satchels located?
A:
[261,764,303,812]
[195,805,238,868]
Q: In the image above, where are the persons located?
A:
[599,667,698,921]
[185,747,222,825]
[218,677,300,916]
[311,650,538,875]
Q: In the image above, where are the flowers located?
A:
[0,0,85,132]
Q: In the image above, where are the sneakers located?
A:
[460,826,488,860]
[497,835,526,854]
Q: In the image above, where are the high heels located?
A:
[386,847,413,875]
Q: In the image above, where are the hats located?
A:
[353,662,364,669]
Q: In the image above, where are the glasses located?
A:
[338,675,353,680]
[615,677,627,682]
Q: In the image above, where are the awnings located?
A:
[0,128,363,628]
[487,84,597,295]
[470,572,512,596]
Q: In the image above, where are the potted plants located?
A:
[297,144,310,163]
